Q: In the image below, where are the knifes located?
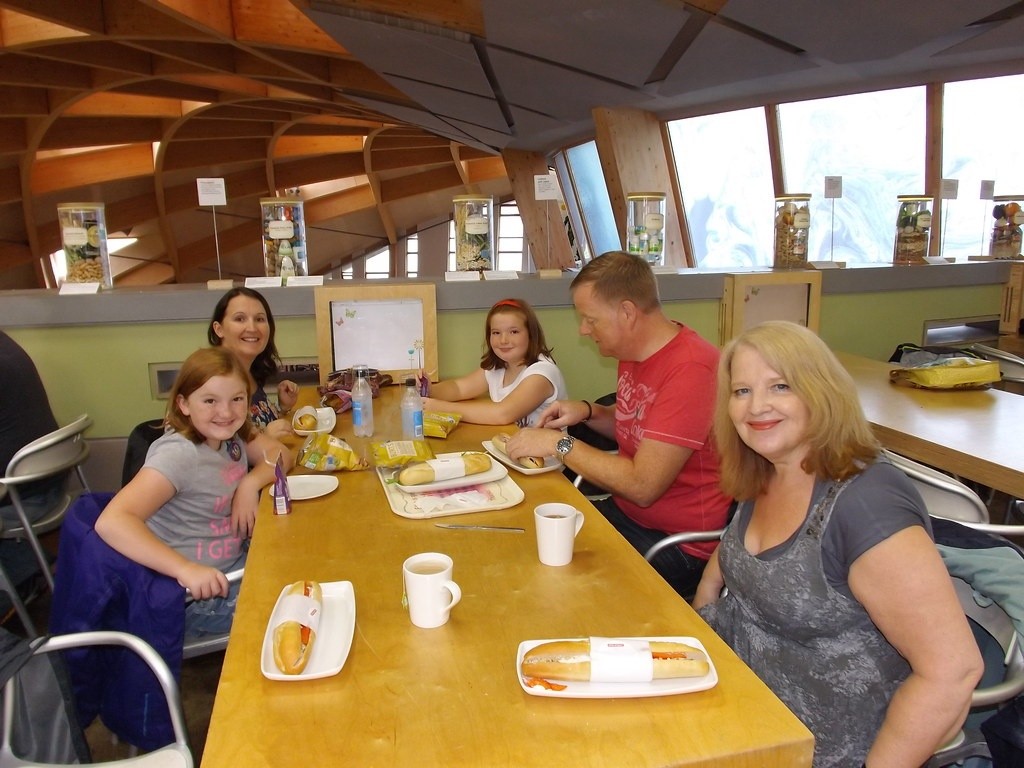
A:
[435,523,525,534]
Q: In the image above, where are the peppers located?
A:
[993,202,1021,219]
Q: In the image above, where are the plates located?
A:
[269,474,339,500]
[392,451,508,493]
[482,441,563,475]
[516,637,719,699]
[291,407,336,435]
[260,580,356,681]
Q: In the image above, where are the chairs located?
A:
[0,631,196,767]
[64,492,244,758]
[0,413,97,637]
[879,442,1024,768]
[573,448,734,566]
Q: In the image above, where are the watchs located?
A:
[556,435,575,462]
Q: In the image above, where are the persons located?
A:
[0,332,71,618]
[505,251,735,594]
[415,300,569,472]
[690,319,984,768]
[208,288,299,441]
[94,349,294,634]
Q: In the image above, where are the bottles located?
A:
[400,379,424,440]
[351,370,374,437]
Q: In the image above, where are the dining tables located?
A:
[196,384,815,768]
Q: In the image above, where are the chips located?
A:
[324,395,345,409]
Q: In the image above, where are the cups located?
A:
[452,194,493,280]
[56,202,113,289]
[774,193,812,268]
[534,503,584,566]
[258,196,308,285]
[625,192,667,268]
[350,363,371,389]
[989,195,1024,260]
[403,552,461,628]
[893,195,935,265]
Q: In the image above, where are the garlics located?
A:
[777,203,806,214]
[994,209,1024,228]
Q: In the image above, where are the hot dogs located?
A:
[387,453,491,485]
[492,432,545,469]
[274,580,324,676]
[521,639,710,679]
[294,405,317,431]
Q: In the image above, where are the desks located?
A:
[833,348,1024,501]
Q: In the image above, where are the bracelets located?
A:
[582,400,592,422]
